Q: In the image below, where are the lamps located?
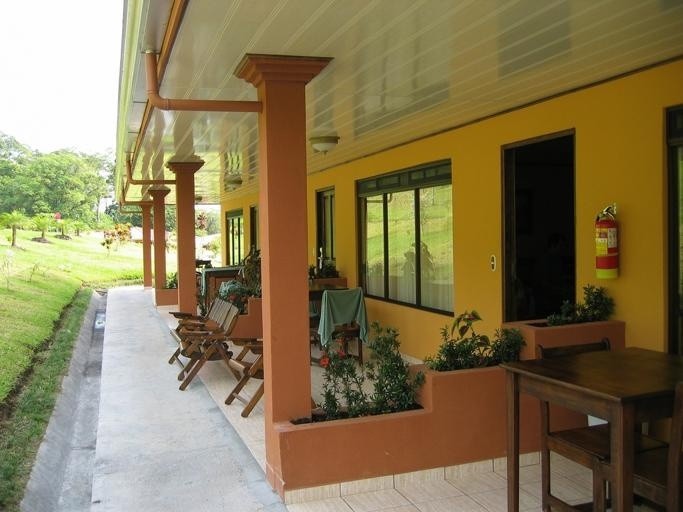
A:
[226,180,242,190]
[195,198,203,204]
[309,136,340,155]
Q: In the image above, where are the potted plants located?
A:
[198,247,347,339]
[166,272,178,304]
[273,283,626,505]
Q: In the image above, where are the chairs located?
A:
[601,446,683,512]
[225,345,317,418]
[168,298,241,390]
[534,338,667,512]
[310,287,362,367]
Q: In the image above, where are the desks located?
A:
[499,347,683,512]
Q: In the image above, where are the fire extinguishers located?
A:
[595,202,618,269]
